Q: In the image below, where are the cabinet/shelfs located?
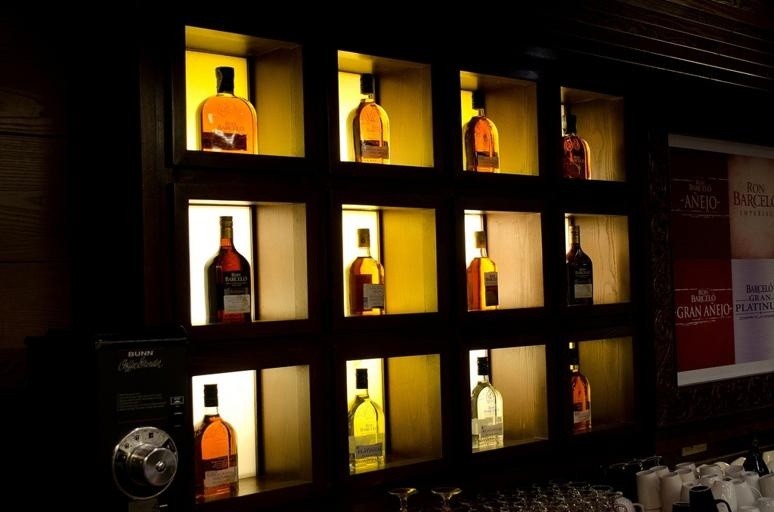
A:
[141,24,328,512]
[556,86,657,471]
[458,70,556,483]
[328,48,458,509]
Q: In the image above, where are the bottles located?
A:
[466,230,498,310]
[563,114,590,178]
[348,228,386,316]
[470,356,504,449]
[348,367,385,474]
[346,72,390,165]
[568,348,592,434]
[463,89,499,173]
[191,384,239,501]
[567,225,594,306]
[203,215,251,323]
[195,66,258,154]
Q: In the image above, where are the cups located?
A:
[610,462,629,475]
[730,479,762,506]
[461,481,628,511]
[759,473,774,498]
[387,488,417,512]
[714,479,738,511]
[689,485,732,512]
[662,472,684,511]
[648,456,662,465]
[700,464,724,480]
[701,473,719,488]
[725,465,745,479]
[741,471,762,499]
[763,451,773,473]
[633,458,645,471]
[675,468,694,485]
[741,507,760,512]
[431,486,462,512]
[675,461,696,476]
[636,470,661,509]
[651,464,669,476]
